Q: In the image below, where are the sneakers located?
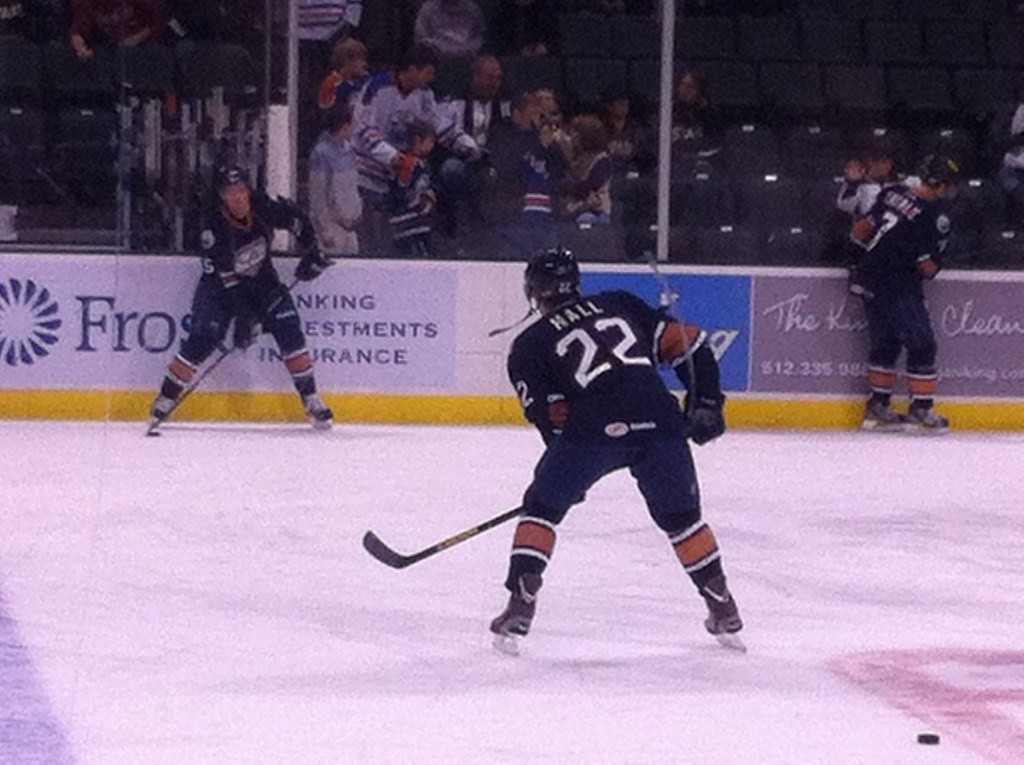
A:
[303,394,334,431]
[697,572,749,659]
[489,575,542,659]
[857,405,914,435]
[148,397,173,429]
[900,400,952,435]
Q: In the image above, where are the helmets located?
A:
[215,164,248,190]
[523,245,581,295]
[919,150,961,199]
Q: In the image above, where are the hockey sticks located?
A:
[145,278,302,438]
[361,423,692,569]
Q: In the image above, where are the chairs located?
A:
[0,0,1024,271]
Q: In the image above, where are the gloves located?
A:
[294,252,332,281]
[685,393,728,446]
[232,310,264,350]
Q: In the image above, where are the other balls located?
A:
[917,733,940,746]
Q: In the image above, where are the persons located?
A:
[488,244,748,637]
[149,161,335,425]
[0,0,1024,274]
[848,151,971,429]
